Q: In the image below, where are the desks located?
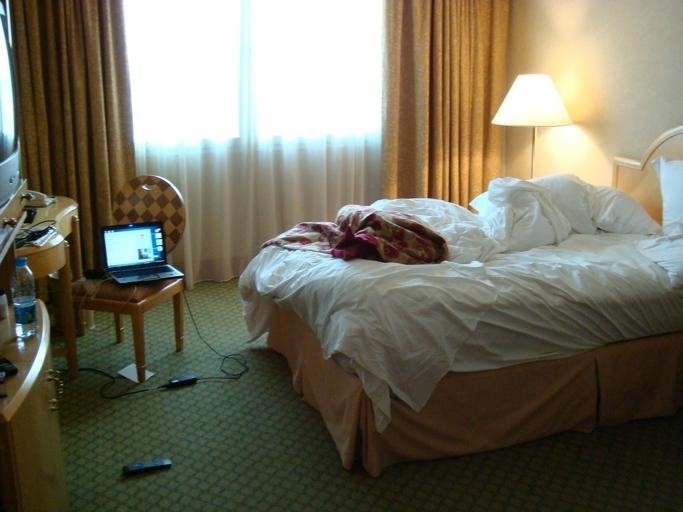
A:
[14,195,86,379]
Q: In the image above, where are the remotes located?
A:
[122,459,172,477]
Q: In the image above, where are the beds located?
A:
[267,124,683,478]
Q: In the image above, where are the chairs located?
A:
[70,175,187,382]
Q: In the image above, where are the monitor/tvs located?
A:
[0,1,22,211]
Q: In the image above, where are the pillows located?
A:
[469,156,683,237]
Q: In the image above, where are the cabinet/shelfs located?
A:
[0,298,67,512]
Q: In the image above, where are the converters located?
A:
[168,372,198,388]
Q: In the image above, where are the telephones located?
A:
[23,189,54,207]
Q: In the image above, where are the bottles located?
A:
[0,288,9,321]
[15,336,37,359]
[8,256,38,340]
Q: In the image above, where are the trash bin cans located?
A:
[48,270,85,337]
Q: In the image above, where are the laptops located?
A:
[102,219,185,285]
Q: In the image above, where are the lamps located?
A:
[491,73,570,180]
